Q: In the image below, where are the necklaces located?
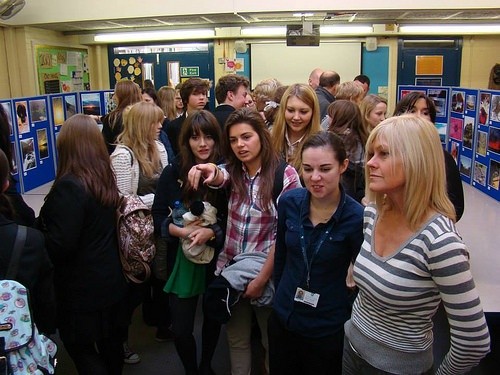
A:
[309,198,340,221]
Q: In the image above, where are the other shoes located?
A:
[121,347,142,364]
[155,327,174,342]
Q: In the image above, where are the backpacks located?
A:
[112,174,155,287]
[0,225,57,375]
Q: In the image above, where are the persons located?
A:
[0,150,61,375]
[149,109,236,375]
[393,91,465,223]
[0,101,35,228]
[491,95,500,122]
[342,112,492,375]
[268,83,324,188]
[108,101,181,365]
[266,130,368,375]
[210,74,249,151]
[17,104,30,134]
[97,68,386,149]
[326,100,372,203]
[38,114,130,375]
[186,108,305,375]
[166,78,210,156]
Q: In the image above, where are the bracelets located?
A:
[200,163,218,183]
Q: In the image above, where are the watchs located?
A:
[209,234,216,240]
